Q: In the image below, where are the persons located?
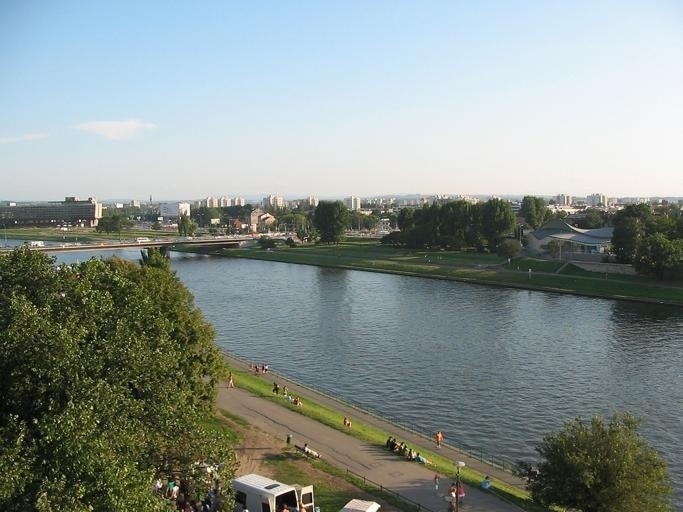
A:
[385,435,431,464]
[154,473,222,512]
[433,473,465,512]
[226,371,234,389]
[435,431,443,448]
[303,443,309,453]
[481,476,491,489]
[273,381,303,406]
[344,416,352,427]
[242,505,250,512]
[249,361,269,376]
[278,502,321,512]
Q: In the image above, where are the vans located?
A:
[231,474,315,512]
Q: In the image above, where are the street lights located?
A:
[452,461,465,512]
